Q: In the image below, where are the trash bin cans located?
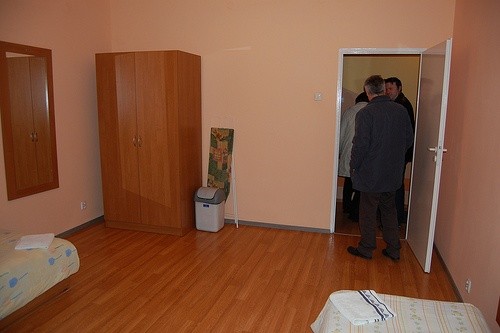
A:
[194,187,226,232]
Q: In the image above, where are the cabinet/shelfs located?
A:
[7,56,55,189]
[95,50,203,236]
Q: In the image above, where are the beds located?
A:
[311,290,490,333]
[0,229,80,321]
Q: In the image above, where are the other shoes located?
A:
[381,249,400,261]
[346,246,370,259]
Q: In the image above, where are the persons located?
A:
[347,75,413,260]
[339,77,415,225]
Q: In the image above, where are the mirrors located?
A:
[0,40,60,201]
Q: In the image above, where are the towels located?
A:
[13,232,56,251]
[328,287,397,327]
